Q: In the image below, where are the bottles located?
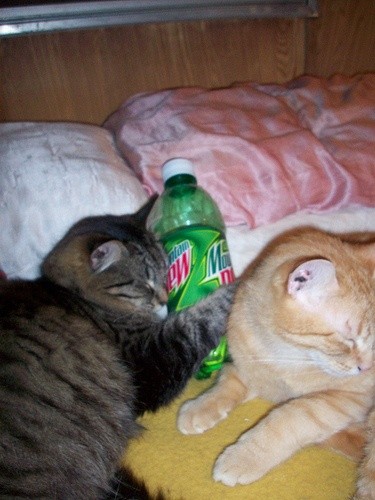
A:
[150,158,237,380]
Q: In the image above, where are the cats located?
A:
[174,225,373,500]
[0,192,244,500]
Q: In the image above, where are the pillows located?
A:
[1,121,149,286]
[102,70,375,228]
